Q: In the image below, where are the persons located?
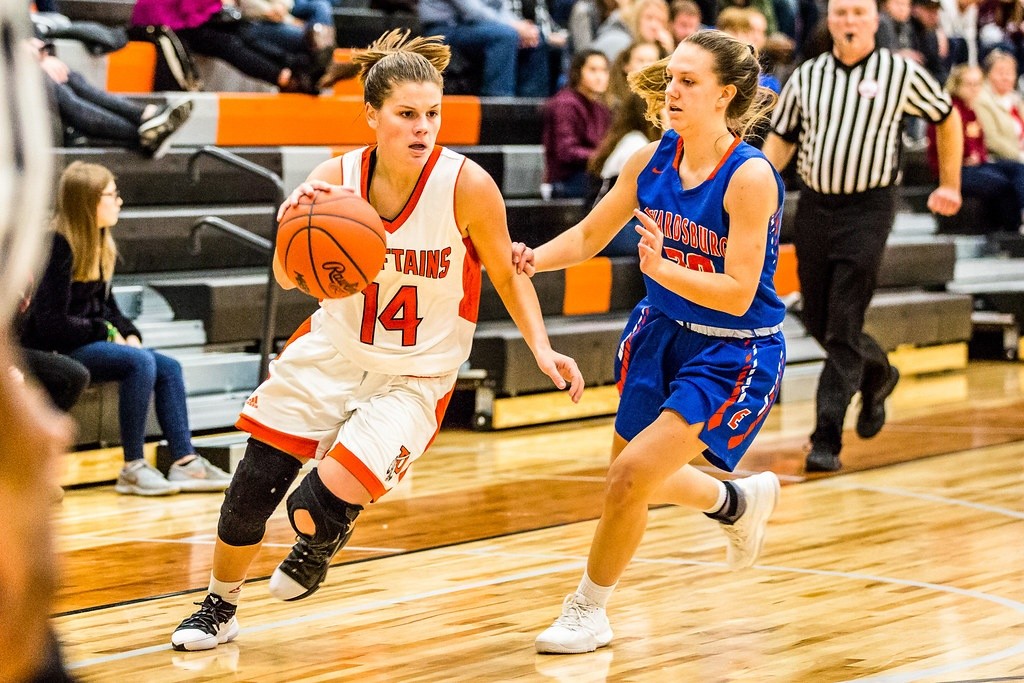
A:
[510,29,787,654]
[412,0,1024,367]
[172,25,585,652]
[0,0,363,682]
[761,0,963,472]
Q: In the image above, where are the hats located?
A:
[912,0,944,10]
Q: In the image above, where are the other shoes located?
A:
[51,487,64,504]
[280,44,333,98]
[317,62,361,91]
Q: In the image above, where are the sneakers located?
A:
[805,446,838,471]
[720,472,780,572]
[535,653,613,683]
[171,593,240,651]
[856,366,899,439]
[138,99,194,160]
[535,592,613,653]
[269,523,359,601]
[168,455,234,490]
[116,458,179,496]
[171,642,241,673]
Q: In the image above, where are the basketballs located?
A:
[276,187,387,298]
